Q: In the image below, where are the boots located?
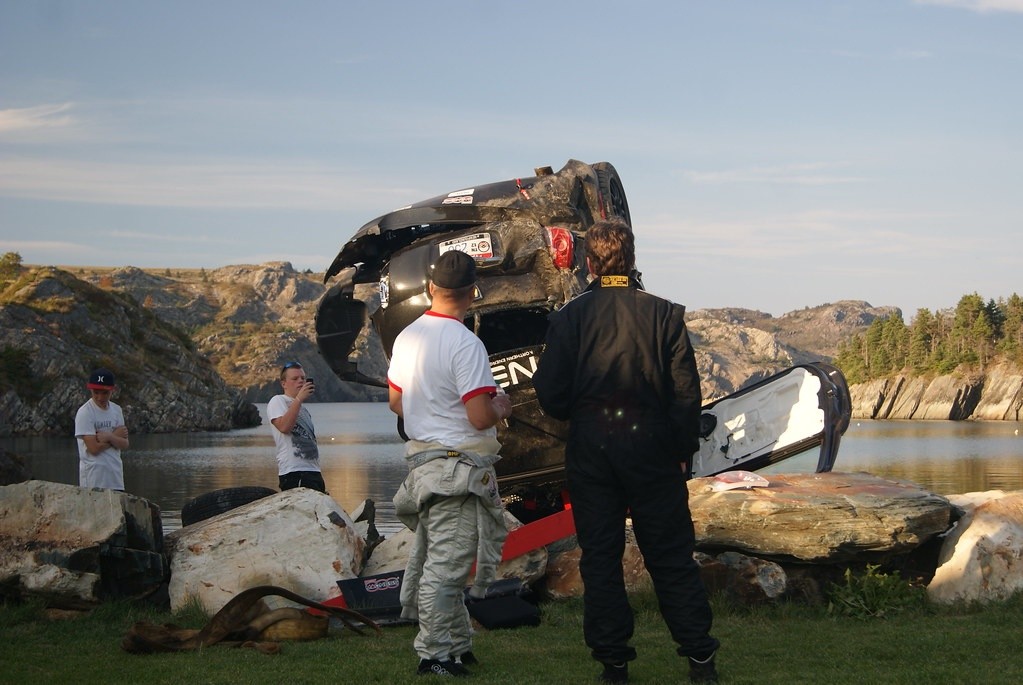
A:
[599,665,628,685]
[686,652,719,685]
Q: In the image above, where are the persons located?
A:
[75,369,129,491]
[389,250,512,678]
[532,220,720,685]
[267,362,326,493]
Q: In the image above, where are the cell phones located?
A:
[306,377,314,393]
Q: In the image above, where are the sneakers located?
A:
[416,657,476,679]
[448,651,478,666]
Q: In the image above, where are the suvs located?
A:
[313,158,852,525]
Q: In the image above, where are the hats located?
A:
[87,368,115,389]
[432,250,477,288]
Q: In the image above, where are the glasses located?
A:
[280,361,302,377]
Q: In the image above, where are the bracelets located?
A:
[295,394,301,403]
